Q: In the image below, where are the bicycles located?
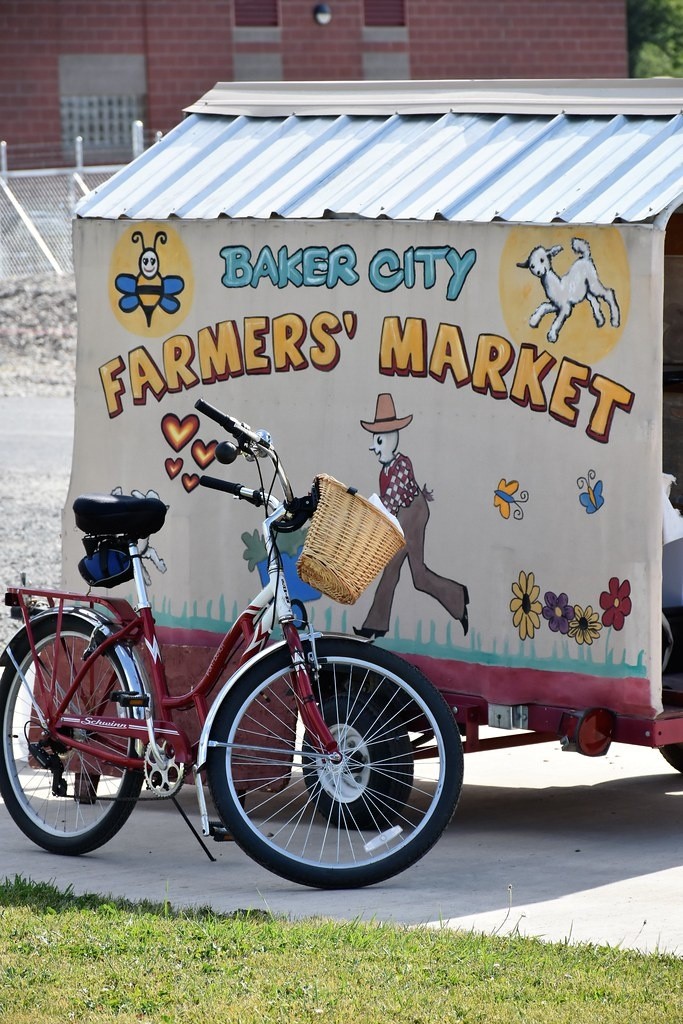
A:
[0,398,464,891]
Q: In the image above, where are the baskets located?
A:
[298,475,407,605]
